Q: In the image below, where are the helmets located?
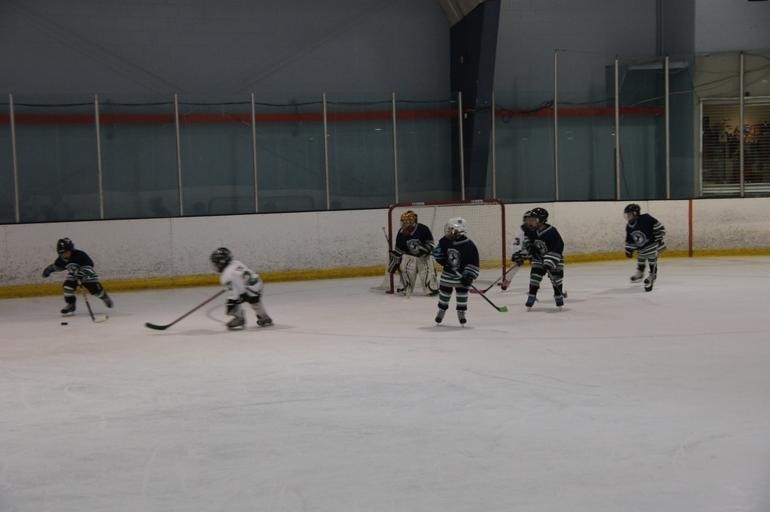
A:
[401,210,417,232]
[56,237,74,254]
[622,203,641,216]
[523,208,549,223]
[210,248,233,270]
[444,217,468,240]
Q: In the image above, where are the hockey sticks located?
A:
[145,290,228,329]
[454,269,508,312]
[469,263,517,294]
[76,280,108,323]
[382,226,406,293]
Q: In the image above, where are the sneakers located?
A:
[256,317,272,324]
[396,287,414,295]
[630,272,644,280]
[99,290,111,308]
[644,272,656,283]
[226,317,245,327]
[555,298,564,306]
[435,311,446,322]
[456,310,467,324]
[525,295,536,307]
[60,302,74,312]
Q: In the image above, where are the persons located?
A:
[210,246,273,329]
[501,211,535,289]
[432,217,480,324]
[43,238,113,315]
[389,211,440,296]
[623,203,666,291]
[526,207,565,307]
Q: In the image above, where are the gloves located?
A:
[462,277,472,287]
[538,265,552,277]
[225,300,236,313]
[526,242,539,256]
[42,267,51,278]
